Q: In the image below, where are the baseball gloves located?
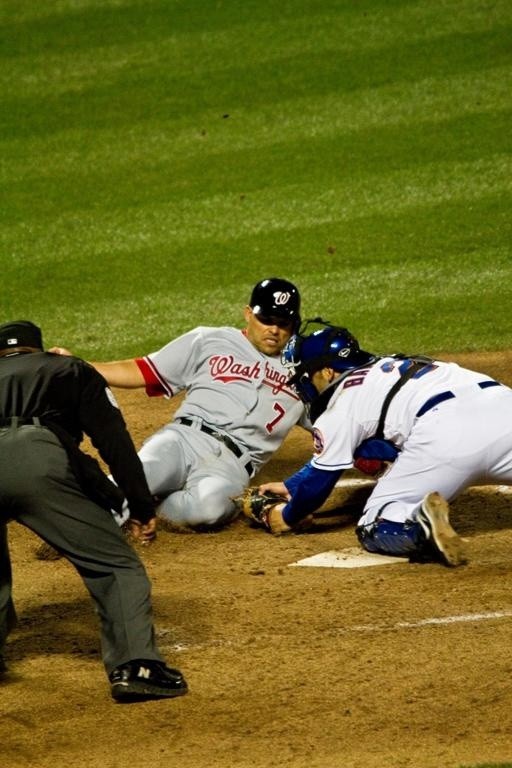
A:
[230,486,291,534]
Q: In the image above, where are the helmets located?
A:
[249,278,300,319]
[281,318,380,404]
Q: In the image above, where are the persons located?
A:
[230,324,511,567]
[0,319,189,701]
[39,276,314,561]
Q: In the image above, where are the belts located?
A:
[180,418,253,476]
[416,381,500,419]
[1,418,48,427]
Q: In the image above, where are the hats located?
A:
[0,321,42,349]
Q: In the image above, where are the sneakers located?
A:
[412,493,466,566]
[109,659,187,701]
